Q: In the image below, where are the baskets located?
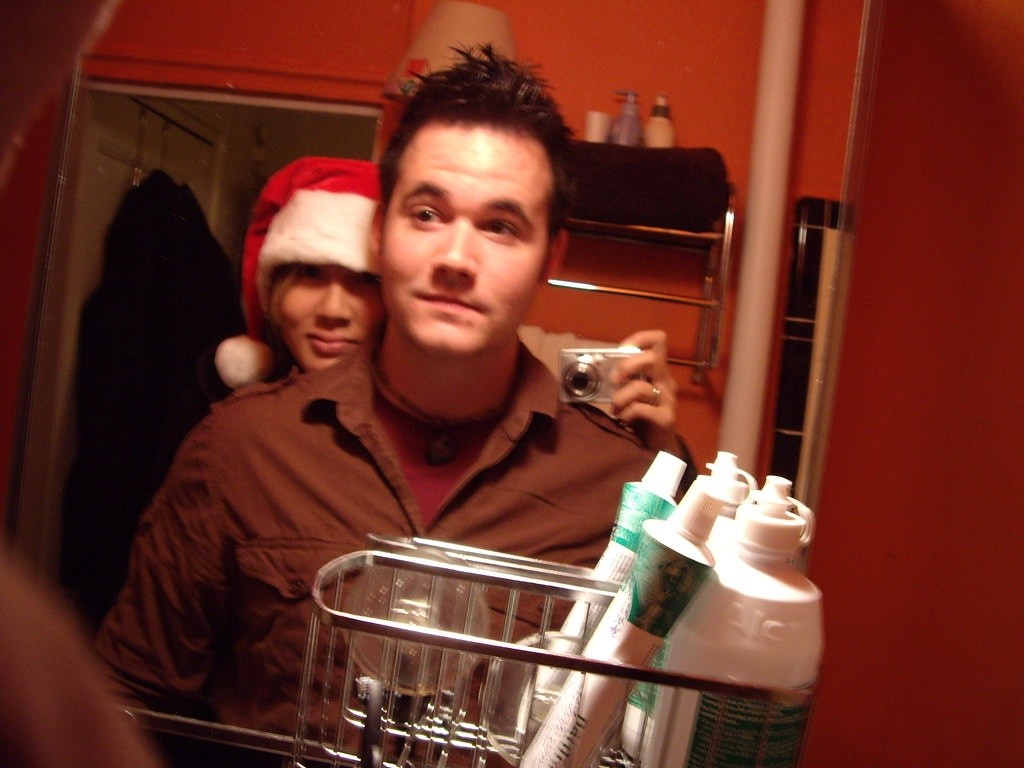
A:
[292,545,811,768]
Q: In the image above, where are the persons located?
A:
[91,42,698,768]
[0,544,161,768]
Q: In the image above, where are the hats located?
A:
[214,155,384,389]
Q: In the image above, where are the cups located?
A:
[583,111,610,143]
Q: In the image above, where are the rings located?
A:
[650,385,661,405]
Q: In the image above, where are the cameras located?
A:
[555,345,648,403]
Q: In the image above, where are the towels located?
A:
[568,143,736,251]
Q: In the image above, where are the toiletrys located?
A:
[581,85,688,147]
[518,475,726,768]
[519,448,687,736]
